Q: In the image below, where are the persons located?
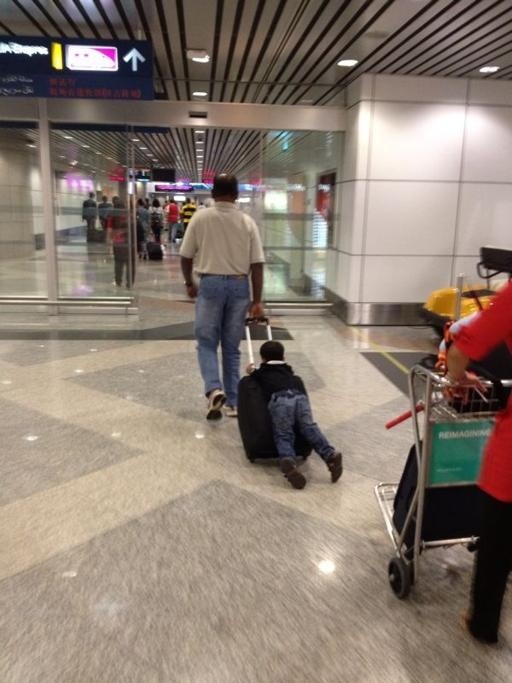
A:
[82,190,215,262]
[444,276,511,645]
[245,340,343,490]
[104,197,136,292]
[178,173,263,421]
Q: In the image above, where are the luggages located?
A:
[392,440,477,560]
[238,318,313,462]
[147,242,162,261]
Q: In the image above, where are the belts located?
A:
[200,274,249,278]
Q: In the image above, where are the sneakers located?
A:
[207,389,227,420]
[280,460,306,489]
[327,452,341,483]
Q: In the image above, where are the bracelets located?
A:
[183,280,194,288]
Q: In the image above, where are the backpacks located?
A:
[151,206,162,234]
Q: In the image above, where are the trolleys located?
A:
[138,232,154,259]
[372,362,512,599]
